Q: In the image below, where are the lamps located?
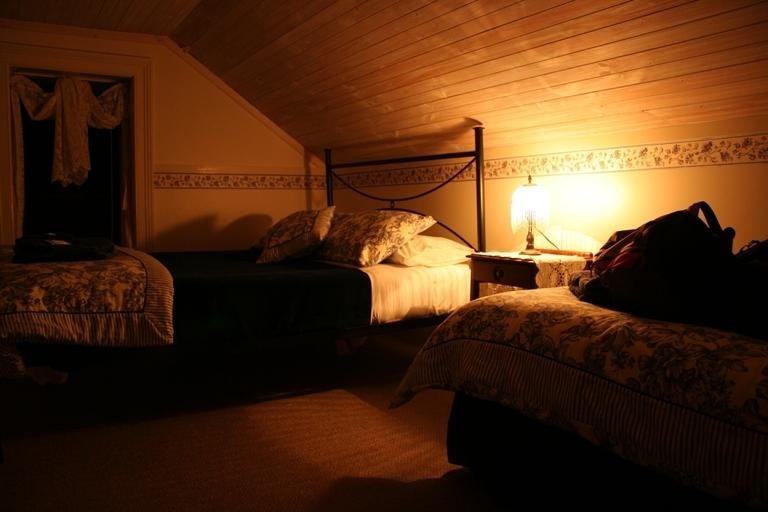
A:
[510,175,551,255]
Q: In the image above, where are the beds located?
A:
[1,128,486,437]
[387,285,768,512]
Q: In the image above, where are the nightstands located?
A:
[466,252,593,301]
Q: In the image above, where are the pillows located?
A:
[313,208,437,267]
[256,206,338,264]
[386,234,475,267]
